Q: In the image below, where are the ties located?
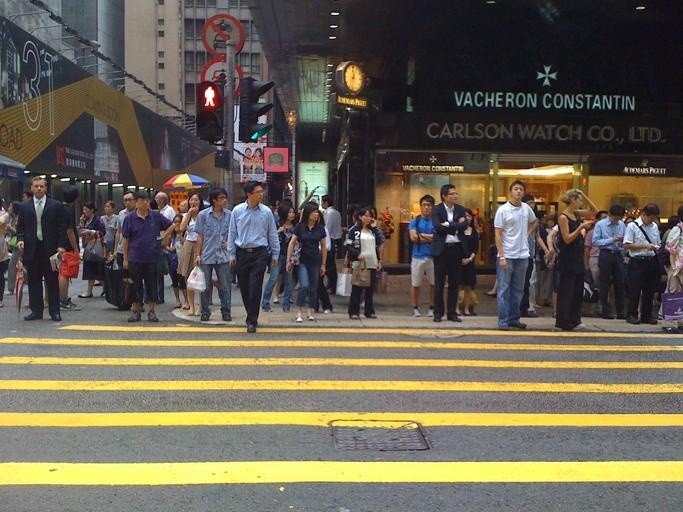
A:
[35,201,43,241]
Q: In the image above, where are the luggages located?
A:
[102,269,122,308]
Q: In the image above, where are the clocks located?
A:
[332,58,368,97]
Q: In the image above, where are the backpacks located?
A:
[656,225,682,266]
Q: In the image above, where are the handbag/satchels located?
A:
[119,278,135,306]
[351,259,371,288]
[661,272,683,320]
[335,267,352,297]
[155,249,170,274]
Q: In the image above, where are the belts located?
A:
[245,248,253,253]
[446,242,455,247]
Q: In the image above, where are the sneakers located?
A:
[59,297,80,311]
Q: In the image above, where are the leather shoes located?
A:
[128,283,376,322]
[498,303,683,334]
[79,294,94,299]
[247,323,256,333]
[484,290,497,297]
[412,305,476,322]
[24,313,42,320]
[50,310,61,321]
[93,282,102,285]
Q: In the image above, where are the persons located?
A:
[485,195,585,317]
[494,182,538,331]
[0,174,233,322]
[253,148,264,173]
[584,203,683,333]
[409,185,478,322]
[556,190,598,331]
[264,196,384,321]
[243,147,253,173]
[227,181,280,332]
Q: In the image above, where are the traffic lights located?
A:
[239,77,275,141]
[195,81,224,141]
